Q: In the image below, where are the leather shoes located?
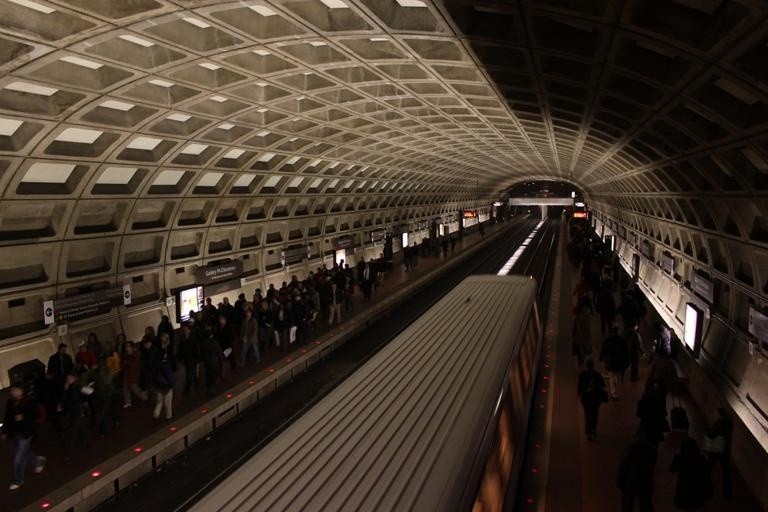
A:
[111,422,119,433]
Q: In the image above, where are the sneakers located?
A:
[9,484,18,489]
[124,402,132,408]
[35,466,42,472]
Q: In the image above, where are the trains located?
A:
[174,275,543,512]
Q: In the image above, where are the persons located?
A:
[4,225,470,491]
[566,218,733,512]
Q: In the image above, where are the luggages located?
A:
[669,385,690,431]
